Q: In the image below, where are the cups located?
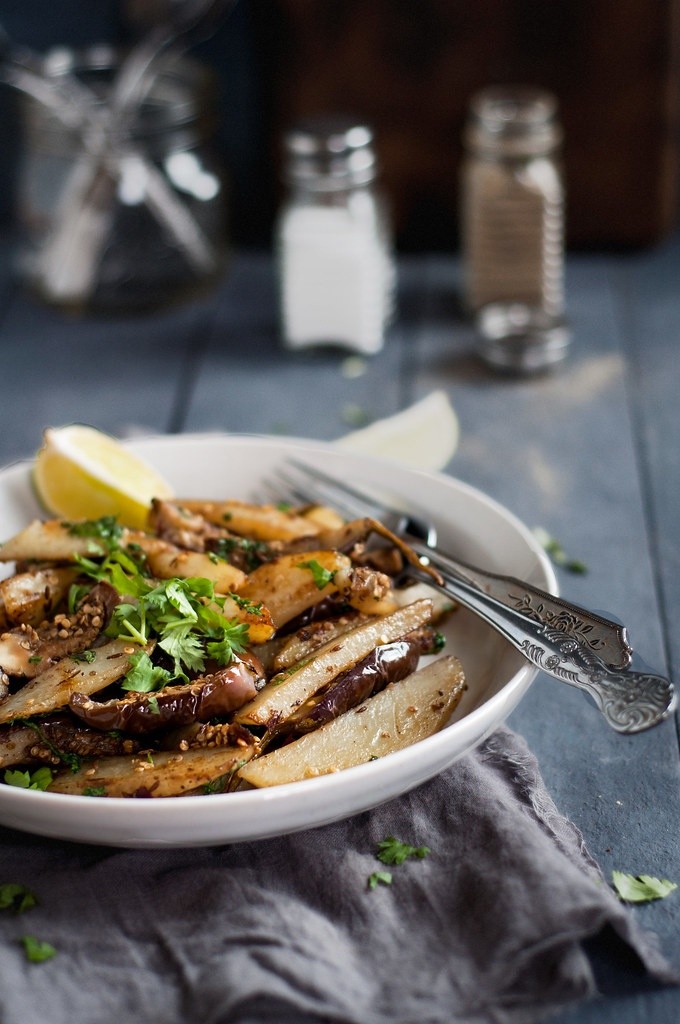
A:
[21,44,227,314]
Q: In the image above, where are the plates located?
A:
[0,433,559,849]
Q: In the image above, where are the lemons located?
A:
[34,423,176,526]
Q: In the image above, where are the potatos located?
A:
[0,497,465,798]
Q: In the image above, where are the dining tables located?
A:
[0,233,680,1024]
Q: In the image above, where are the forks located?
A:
[260,457,634,669]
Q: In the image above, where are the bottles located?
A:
[277,115,399,355]
[460,86,566,322]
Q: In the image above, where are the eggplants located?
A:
[280,627,420,736]
[0,720,70,770]
[70,645,264,729]
